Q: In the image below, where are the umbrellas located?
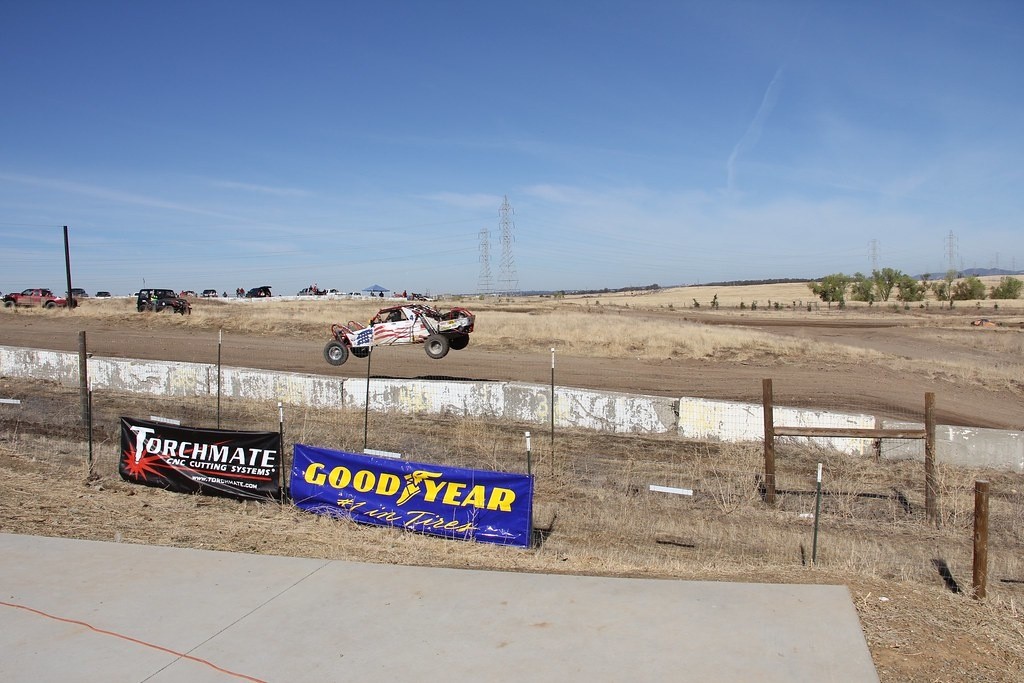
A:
[363,283,390,297]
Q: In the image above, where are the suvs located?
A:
[245,285,272,298]
[135,289,191,317]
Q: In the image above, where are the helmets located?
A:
[390,310,401,322]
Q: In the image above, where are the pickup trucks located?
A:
[2,287,68,310]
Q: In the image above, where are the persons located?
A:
[379,291,384,296]
[389,309,401,322]
[308,283,317,293]
[402,289,407,298]
[235,288,245,299]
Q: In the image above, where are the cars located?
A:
[297,288,313,297]
[131,292,145,297]
[323,303,476,363]
[180,291,198,299]
[202,289,219,297]
[95,291,111,297]
[66,288,88,298]
